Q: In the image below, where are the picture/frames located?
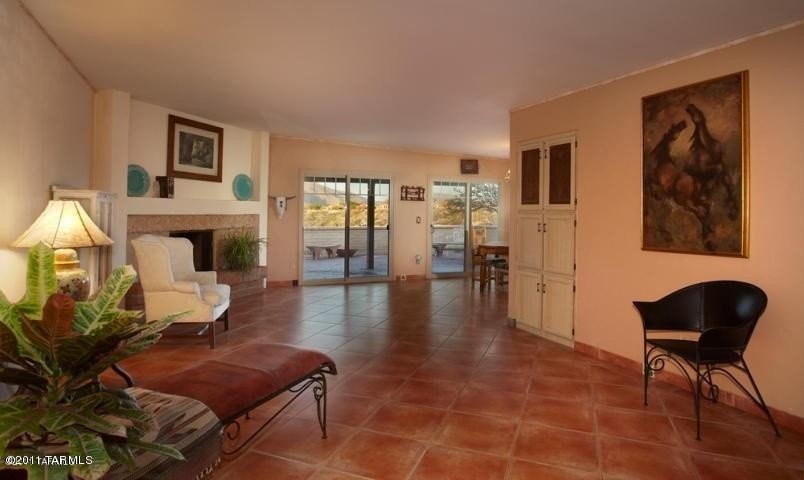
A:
[641,70,751,259]
[461,160,478,174]
[167,115,224,182]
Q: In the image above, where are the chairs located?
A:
[130,234,232,350]
[471,247,508,289]
[632,281,782,441]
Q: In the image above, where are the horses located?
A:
[682,103,740,221]
[651,119,717,238]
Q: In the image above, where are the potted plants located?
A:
[217,225,270,271]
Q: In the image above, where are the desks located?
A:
[480,242,508,296]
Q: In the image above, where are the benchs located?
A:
[433,243,447,256]
[307,245,341,259]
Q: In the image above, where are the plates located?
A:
[232,174,253,201]
[127,164,151,197]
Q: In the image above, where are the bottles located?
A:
[152,181,161,198]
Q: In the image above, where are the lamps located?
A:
[8,199,115,302]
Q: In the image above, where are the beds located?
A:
[22,344,337,479]
